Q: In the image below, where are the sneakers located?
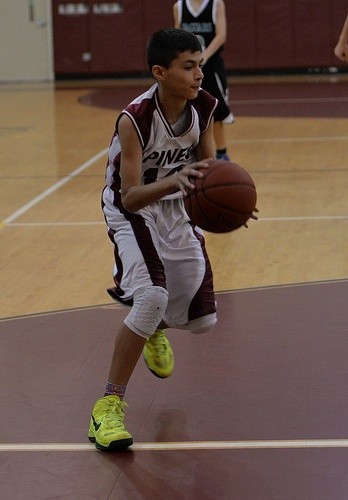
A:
[87,394,133,451]
[216,154,229,161]
[143,330,174,378]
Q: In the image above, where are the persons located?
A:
[88,30,259,451]
[170,0,230,163]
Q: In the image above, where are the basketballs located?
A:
[184,161,257,233]
